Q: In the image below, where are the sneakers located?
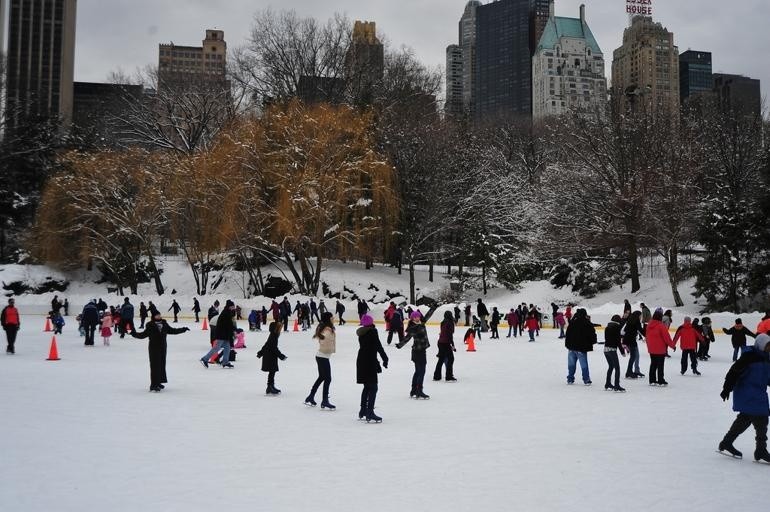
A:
[411,392,427,398]
[693,368,701,375]
[321,400,335,408]
[446,375,456,380]
[754,448,770,463]
[266,386,280,394]
[633,373,645,376]
[658,381,668,385]
[649,380,657,384]
[605,384,613,388]
[306,395,316,405]
[613,385,625,391]
[626,373,637,378]
[201,358,209,368]
[359,409,382,421]
[150,384,165,391]
[718,441,743,456]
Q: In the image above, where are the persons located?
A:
[384,302,414,344]
[200,299,247,369]
[395,302,439,400]
[356,314,389,423]
[303,311,336,411]
[565,298,715,393]
[0,298,20,353]
[49,294,201,346]
[715,332,769,465]
[129,310,191,392]
[432,311,457,383]
[454,296,574,341]
[723,309,769,362]
[256,322,288,396]
[248,296,371,332]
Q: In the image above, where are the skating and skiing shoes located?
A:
[223,363,234,367]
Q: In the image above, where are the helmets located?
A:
[152,307,160,317]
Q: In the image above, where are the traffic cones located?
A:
[209,339,219,364]
[43,318,52,332]
[292,319,298,333]
[126,322,131,330]
[45,337,61,361]
[466,333,477,353]
[202,317,209,331]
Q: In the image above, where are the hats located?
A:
[360,313,374,326]
[410,311,421,319]
